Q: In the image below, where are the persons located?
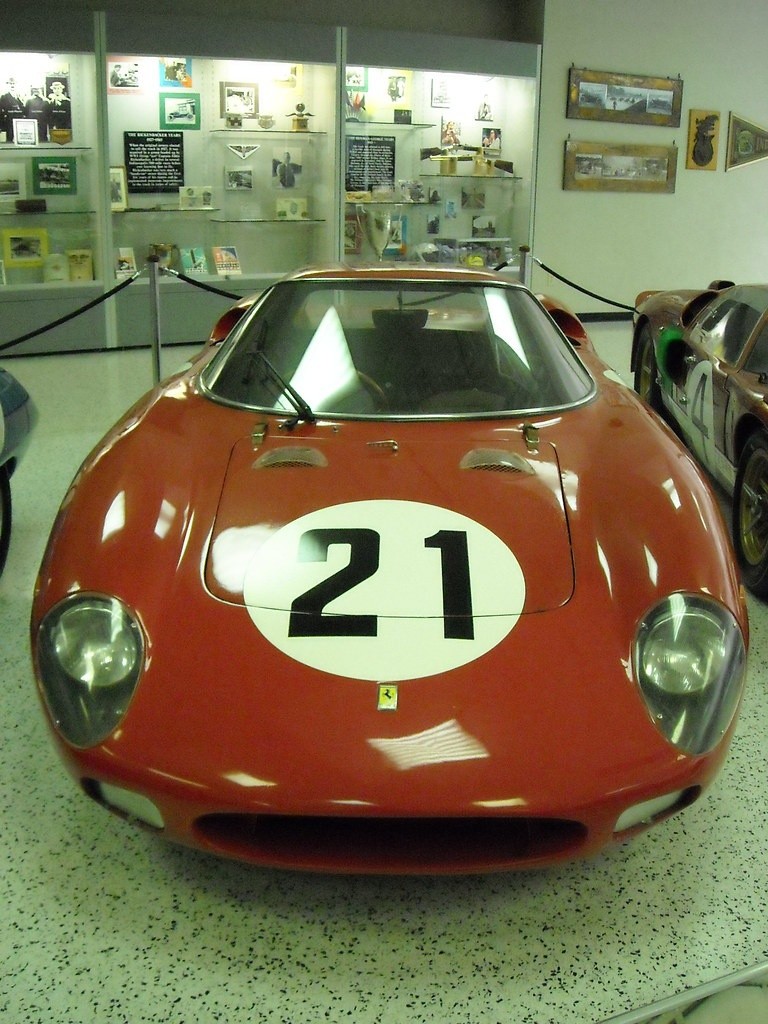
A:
[442,121,461,146]
[0,78,71,142]
[111,178,121,202]
[579,157,591,174]
[431,216,440,233]
[388,77,405,101]
[447,200,456,219]
[227,90,254,112]
[480,94,492,120]
[165,62,186,80]
[185,188,211,208]
[483,247,501,264]
[430,191,440,203]
[482,130,500,149]
[44,167,68,187]
[276,152,295,187]
[400,183,424,202]
[110,64,139,87]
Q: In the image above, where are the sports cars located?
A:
[30,264,751,875]
[629,280,768,599]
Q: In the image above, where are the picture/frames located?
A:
[3,227,49,268]
[12,118,38,146]
[158,57,192,88]
[346,66,369,92]
[381,69,413,110]
[158,91,201,130]
[379,215,408,256]
[219,80,259,119]
[108,164,128,211]
[31,156,78,195]
[566,67,683,128]
[107,55,146,95]
[345,213,366,254]
[562,140,678,194]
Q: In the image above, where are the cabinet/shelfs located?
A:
[0,145,97,217]
[344,118,523,205]
[110,129,328,224]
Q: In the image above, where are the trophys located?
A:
[355,204,404,268]
[286,103,315,129]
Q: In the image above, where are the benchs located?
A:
[284,326,527,411]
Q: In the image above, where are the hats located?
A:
[6,78,16,84]
[30,81,44,89]
[11,243,29,251]
[50,82,65,90]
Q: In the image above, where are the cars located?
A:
[648,96,672,111]
[580,88,603,104]
[167,105,194,122]
[608,96,635,104]
